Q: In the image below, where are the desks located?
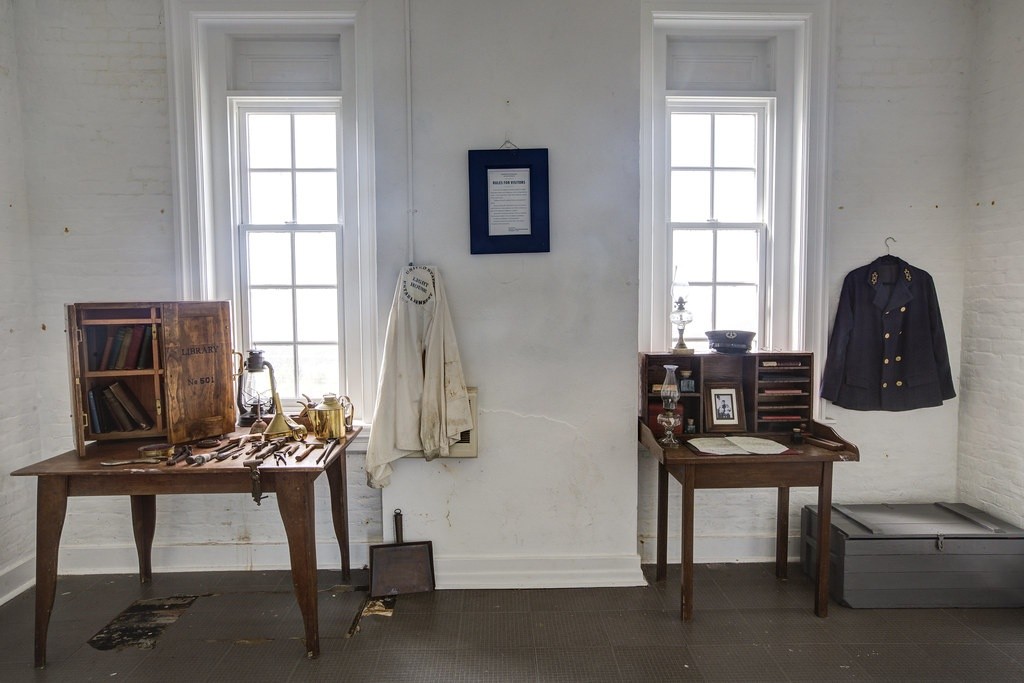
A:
[639,351,859,623]
[10,425,363,669]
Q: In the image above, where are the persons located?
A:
[716,395,733,419]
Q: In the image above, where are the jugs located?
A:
[296,393,354,439]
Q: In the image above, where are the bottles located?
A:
[686,419,696,433]
[680,370,695,392]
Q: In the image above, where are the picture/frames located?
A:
[704,380,747,432]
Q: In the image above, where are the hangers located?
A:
[878,237,897,286]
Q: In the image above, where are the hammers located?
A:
[296,441,325,461]
[166,444,195,466]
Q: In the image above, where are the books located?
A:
[86,325,153,434]
[759,361,803,420]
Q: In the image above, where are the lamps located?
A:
[237,343,276,427]
[657,365,684,448]
[669,266,694,354]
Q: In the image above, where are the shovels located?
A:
[369,508,436,597]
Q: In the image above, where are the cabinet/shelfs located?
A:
[64,302,244,458]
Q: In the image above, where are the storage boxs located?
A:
[648,403,684,434]
[800,502,1024,608]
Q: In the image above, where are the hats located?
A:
[706,331,756,353]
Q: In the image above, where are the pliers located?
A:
[256,436,287,459]
[274,451,288,464]
[316,438,340,464]
[246,440,268,456]
